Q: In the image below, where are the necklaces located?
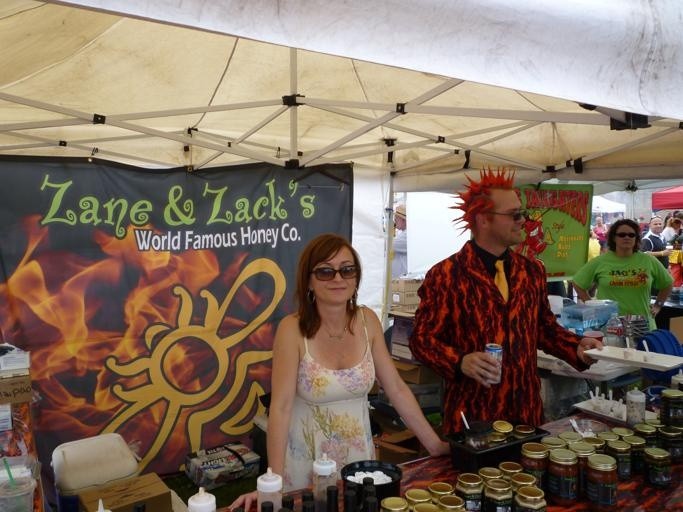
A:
[319,317,349,340]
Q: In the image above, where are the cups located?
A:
[583,330,604,346]
[1,477,38,512]
[666,242,674,252]
[622,348,658,364]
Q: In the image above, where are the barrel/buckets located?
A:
[340,459,403,512]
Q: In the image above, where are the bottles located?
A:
[233,476,380,511]
[671,369,683,388]
[671,285,683,306]
[626,386,645,426]
[605,311,624,347]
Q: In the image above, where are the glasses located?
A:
[614,232,637,238]
[309,265,359,281]
[488,208,530,221]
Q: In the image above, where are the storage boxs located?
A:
[77,471,172,512]
[377,312,440,410]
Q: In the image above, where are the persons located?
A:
[590,208,682,275]
[225,233,453,511]
[407,165,602,440]
[569,218,673,339]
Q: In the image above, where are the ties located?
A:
[494,259,509,303]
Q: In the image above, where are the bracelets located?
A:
[655,300,664,306]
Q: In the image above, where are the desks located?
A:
[214,413,682,511]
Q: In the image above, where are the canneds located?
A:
[381,462,546,512]
[519,423,683,507]
[450,420,536,451]
[660,390,683,426]
[483,343,504,384]
